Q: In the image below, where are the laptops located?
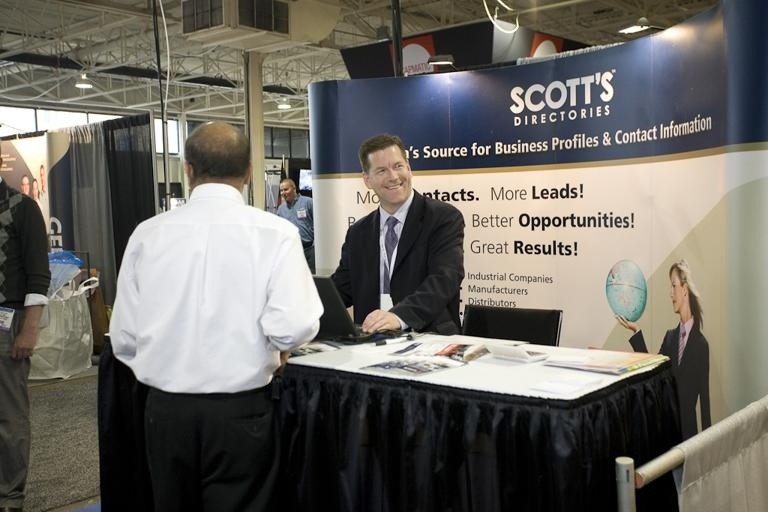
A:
[309,275,404,344]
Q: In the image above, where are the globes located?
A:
[606,260,647,322]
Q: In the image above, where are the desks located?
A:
[95,330,684,511]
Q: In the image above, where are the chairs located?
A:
[460,304,562,346]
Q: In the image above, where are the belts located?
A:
[204,386,269,400]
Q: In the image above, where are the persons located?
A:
[329,130,465,337]
[30,175,40,217]
[16,169,29,197]
[39,160,52,254]
[0,157,53,511]
[276,176,319,276]
[110,119,325,510]
[614,259,714,441]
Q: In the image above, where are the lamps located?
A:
[74,72,93,90]
[615,16,664,36]
[427,52,461,72]
[277,96,291,110]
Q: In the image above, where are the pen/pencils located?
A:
[376,336,411,346]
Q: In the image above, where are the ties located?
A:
[678,325,685,363]
[384,215,400,294]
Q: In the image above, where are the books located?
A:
[545,345,673,374]
